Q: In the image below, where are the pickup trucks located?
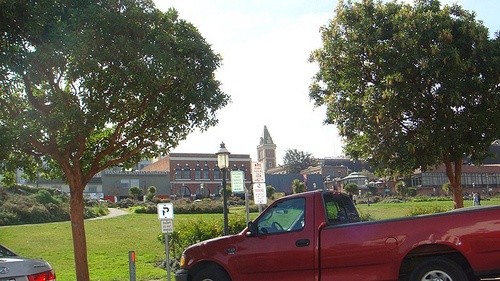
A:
[175,188,500,281]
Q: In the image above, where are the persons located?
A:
[471,191,482,206]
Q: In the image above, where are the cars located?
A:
[0,244,56,281]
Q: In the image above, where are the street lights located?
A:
[215,141,231,236]
